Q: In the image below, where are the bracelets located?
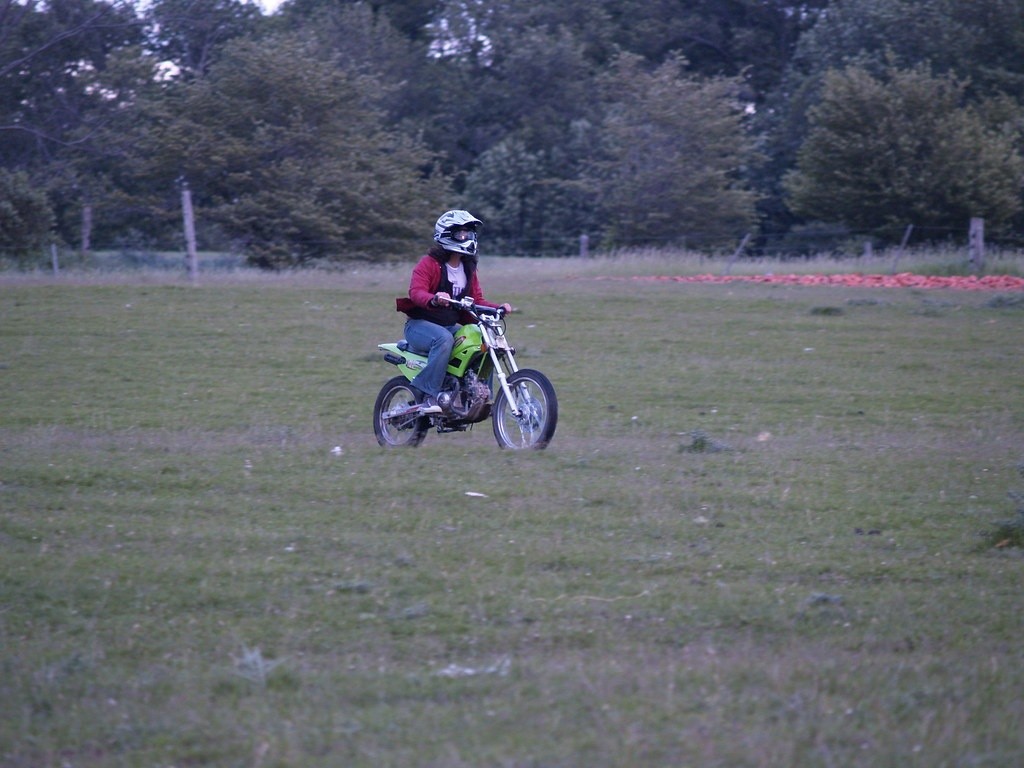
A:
[431,299,438,306]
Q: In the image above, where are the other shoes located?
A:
[420,396,443,413]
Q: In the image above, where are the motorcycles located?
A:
[373,295,559,453]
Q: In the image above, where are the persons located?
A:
[404,210,512,413]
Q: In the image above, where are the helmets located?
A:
[433,210,483,256]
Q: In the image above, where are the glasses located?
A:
[441,228,478,242]
